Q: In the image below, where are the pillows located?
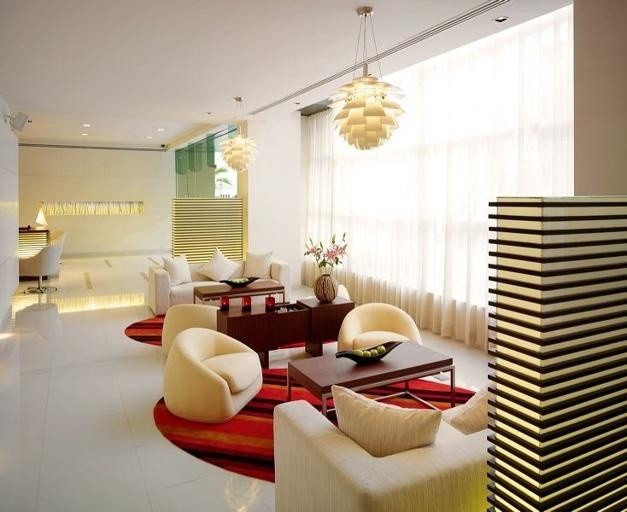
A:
[241,249,274,279]
[198,247,241,282]
[331,384,442,455]
[163,254,192,284]
[443,383,489,433]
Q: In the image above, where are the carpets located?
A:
[154,368,476,482]
[125,314,336,349]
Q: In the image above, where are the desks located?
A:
[298,294,354,356]
[216,302,307,369]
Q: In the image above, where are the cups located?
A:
[265,297,275,313]
[220,294,229,311]
[241,295,251,312]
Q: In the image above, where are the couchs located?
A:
[148,261,291,316]
[273,400,487,512]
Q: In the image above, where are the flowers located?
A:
[305,233,348,273]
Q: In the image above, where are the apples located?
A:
[351,346,386,358]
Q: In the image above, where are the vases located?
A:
[314,274,339,301]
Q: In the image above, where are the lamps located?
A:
[220,96,256,172]
[329,5,403,148]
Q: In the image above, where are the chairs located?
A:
[20,232,65,294]
[338,303,423,351]
[161,303,219,357]
[163,327,263,423]
